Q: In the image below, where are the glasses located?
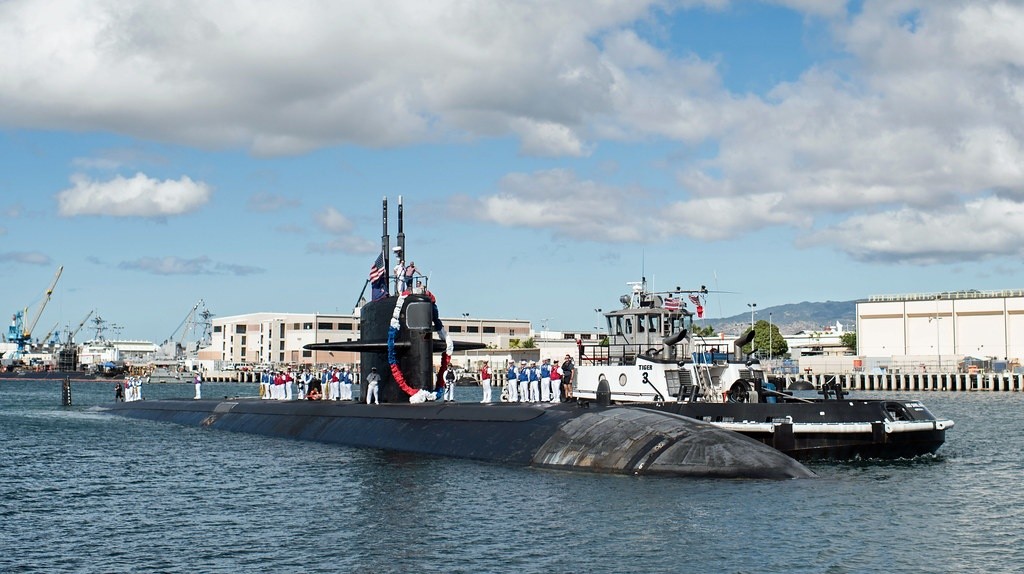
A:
[565,358,568,359]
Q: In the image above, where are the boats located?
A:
[563,270,769,404]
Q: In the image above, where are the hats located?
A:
[554,360,559,363]
[546,357,551,360]
[263,365,377,377]
[448,363,453,366]
[542,358,546,362]
[509,360,515,363]
[530,361,535,364]
[484,359,489,361]
[124,376,141,382]
[195,372,199,374]
[522,360,527,364]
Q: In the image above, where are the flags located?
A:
[664,297,680,310]
[369,250,385,284]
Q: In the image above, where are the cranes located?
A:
[8,266,63,354]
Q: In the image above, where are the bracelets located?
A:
[570,379,573,380]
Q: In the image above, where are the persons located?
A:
[480,361,492,403]
[393,260,425,295]
[507,354,564,403]
[261,365,354,401]
[443,363,457,402]
[366,367,381,404]
[115,375,142,403]
[193,372,202,399]
[561,354,575,403]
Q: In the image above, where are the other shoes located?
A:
[444,396,572,404]
[375,401,379,404]
[194,397,200,399]
